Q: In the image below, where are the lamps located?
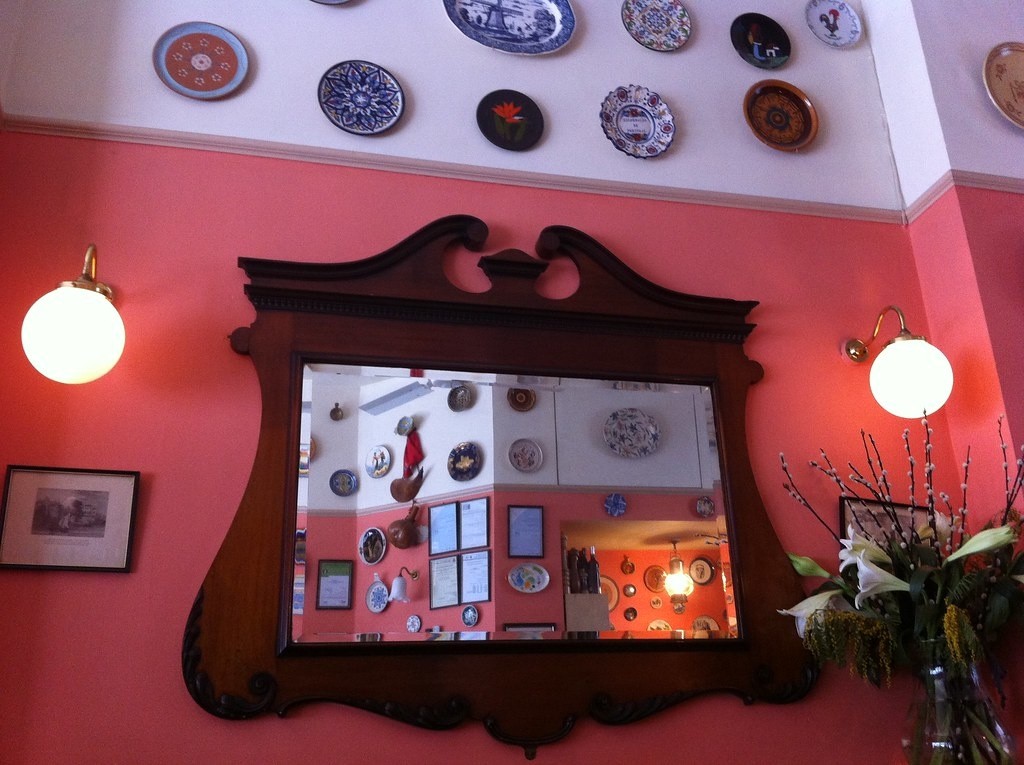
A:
[386,568,420,605]
[663,539,695,609]
[21,244,125,386]
[841,307,953,417]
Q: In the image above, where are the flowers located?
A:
[774,408,1024,765]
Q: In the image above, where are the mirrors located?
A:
[180,213,820,762]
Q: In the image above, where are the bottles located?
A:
[565,546,601,595]
[386,505,419,548]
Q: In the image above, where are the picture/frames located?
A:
[0,464,141,572]
[838,496,930,549]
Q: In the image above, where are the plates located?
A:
[406,616,421,632]
[982,41,1023,130]
[446,441,480,482]
[508,439,543,472]
[301,0,862,157]
[396,417,413,436]
[604,494,625,517]
[507,386,537,412]
[697,496,716,519]
[462,605,478,628]
[357,526,386,565]
[447,386,472,412]
[602,408,662,457]
[329,469,358,497]
[153,22,250,100]
[506,562,551,593]
[365,580,389,613]
[365,444,391,479]
[599,554,724,628]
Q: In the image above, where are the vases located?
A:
[901,637,1017,765]
